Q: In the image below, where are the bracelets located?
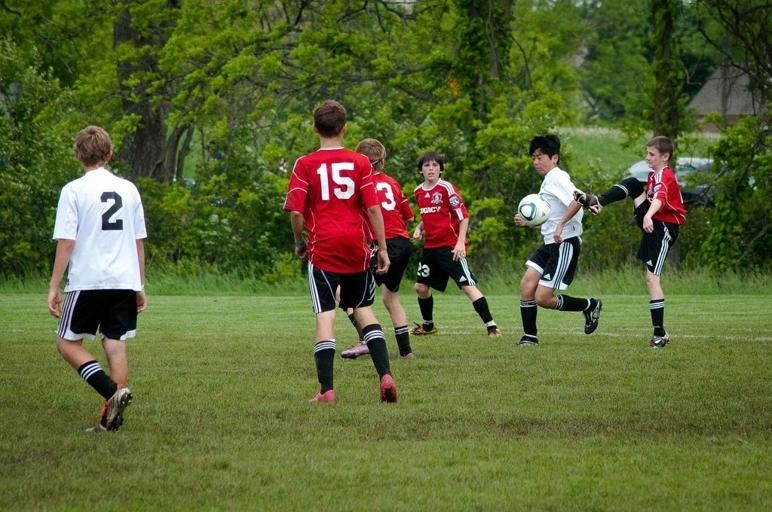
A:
[416,227,423,231]
[295,240,306,248]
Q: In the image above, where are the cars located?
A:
[622,151,755,208]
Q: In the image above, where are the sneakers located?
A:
[380,374,396,402]
[583,297,603,334]
[515,336,540,347]
[85,386,132,434]
[341,341,370,360]
[572,191,602,216]
[411,324,438,336]
[308,388,337,405]
[488,328,502,337]
[650,330,669,350]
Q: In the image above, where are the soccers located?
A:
[518,193,551,227]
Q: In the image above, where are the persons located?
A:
[283,100,397,402]
[46,126,147,432]
[513,136,602,346]
[573,135,687,347]
[342,137,414,360]
[411,151,500,337]
